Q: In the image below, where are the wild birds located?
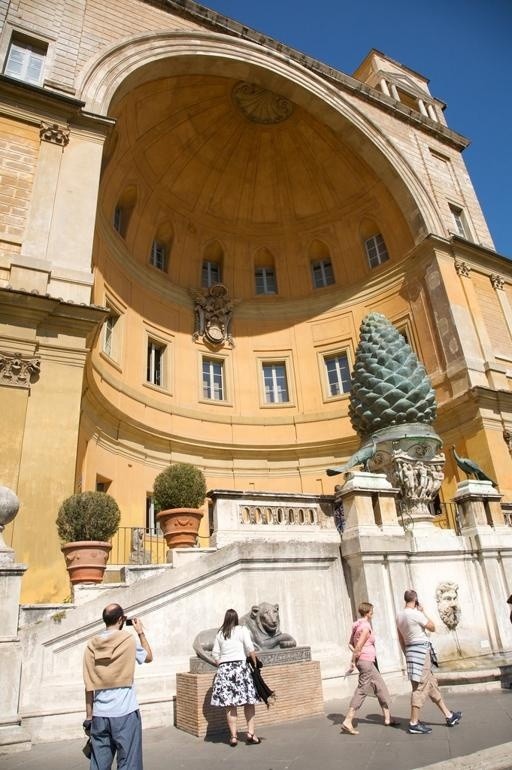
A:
[452,443,498,492]
[327,433,381,476]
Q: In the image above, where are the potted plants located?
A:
[55,489,122,584]
[151,461,210,550]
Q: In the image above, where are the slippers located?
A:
[341,727,359,735]
[385,721,401,726]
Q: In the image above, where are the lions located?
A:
[193,603,296,666]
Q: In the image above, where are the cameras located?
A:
[126,619,135,626]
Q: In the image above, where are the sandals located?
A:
[229,736,239,746]
[246,732,261,745]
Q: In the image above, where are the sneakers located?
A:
[446,711,462,726]
[408,720,432,734]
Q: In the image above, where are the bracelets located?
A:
[138,632,144,637]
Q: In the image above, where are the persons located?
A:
[82,603,152,770]
[341,602,400,735]
[209,609,261,747]
[396,590,462,734]
[398,461,444,501]
[436,581,460,629]
